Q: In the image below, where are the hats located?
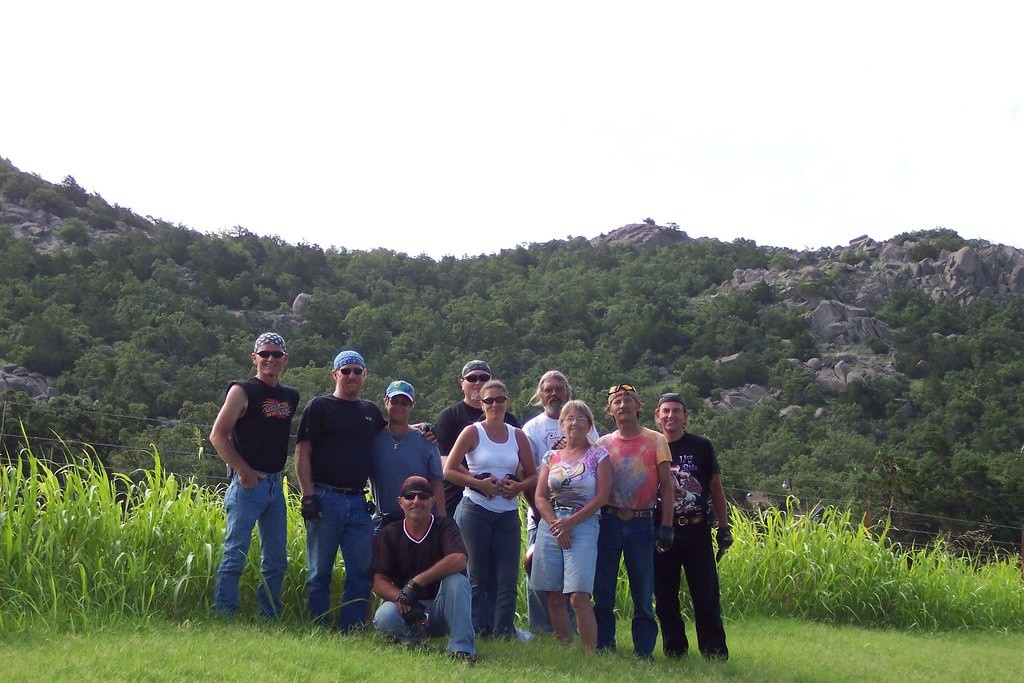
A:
[607,384,642,405]
[255,332,286,355]
[401,475,434,498]
[462,360,491,379]
[385,381,415,404]
[334,351,365,371]
[658,392,685,408]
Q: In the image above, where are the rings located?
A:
[555,528,560,534]
[554,527,556,530]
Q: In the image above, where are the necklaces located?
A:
[386,424,409,450]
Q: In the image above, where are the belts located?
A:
[601,505,653,520]
[654,513,709,526]
[313,483,369,496]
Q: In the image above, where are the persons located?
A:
[443,381,536,637]
[529,400,612,653]
[653,392,733,661]
[209,333,300,620]
[521,370,603,633]
[370,476,476,664]
[366,380,445,556]
[435,362,521,635]
[553,383,674,661]
[293,350,439,635]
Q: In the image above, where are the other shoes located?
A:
[449,651,474,667]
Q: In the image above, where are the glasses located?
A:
[335,368,365,375]
[608,384,637,400]
[481,395,508,404]
[387,398,412,406]
[401,492,433,501]
[255,351,286,359]
[463,374,491,382]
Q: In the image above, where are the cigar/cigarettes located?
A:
[659,548,664,553]
